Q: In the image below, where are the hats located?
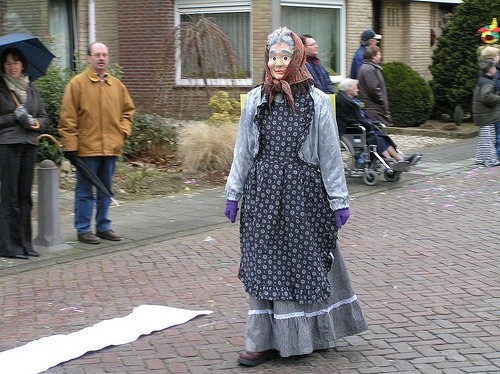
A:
[361,30,382,40]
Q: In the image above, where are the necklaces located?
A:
[480,46,500,159]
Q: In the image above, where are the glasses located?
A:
[91,53,107,57]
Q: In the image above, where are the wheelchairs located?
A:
[327,90,421,185]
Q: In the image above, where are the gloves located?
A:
[14,106,27,119]
[334,209,350,229]
[224,199,239,223]
[21,113,36,127]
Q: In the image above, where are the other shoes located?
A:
[403,153,419,164]
[78,232,101,243]
[11,254,28,260]
[238,352,265,366]
[96,230,121,240]
[26,250,40,257]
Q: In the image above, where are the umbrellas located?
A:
[0,34,61,129]
[34,133,122,208]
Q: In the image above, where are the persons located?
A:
[350,30,383,80]
[472,58,500,168]
[0,46,51,260]
[358,46,393,137]
[334,78,419,170]
[56,40,136,244]
[301,32,335,96]
[221,27,367,368]
